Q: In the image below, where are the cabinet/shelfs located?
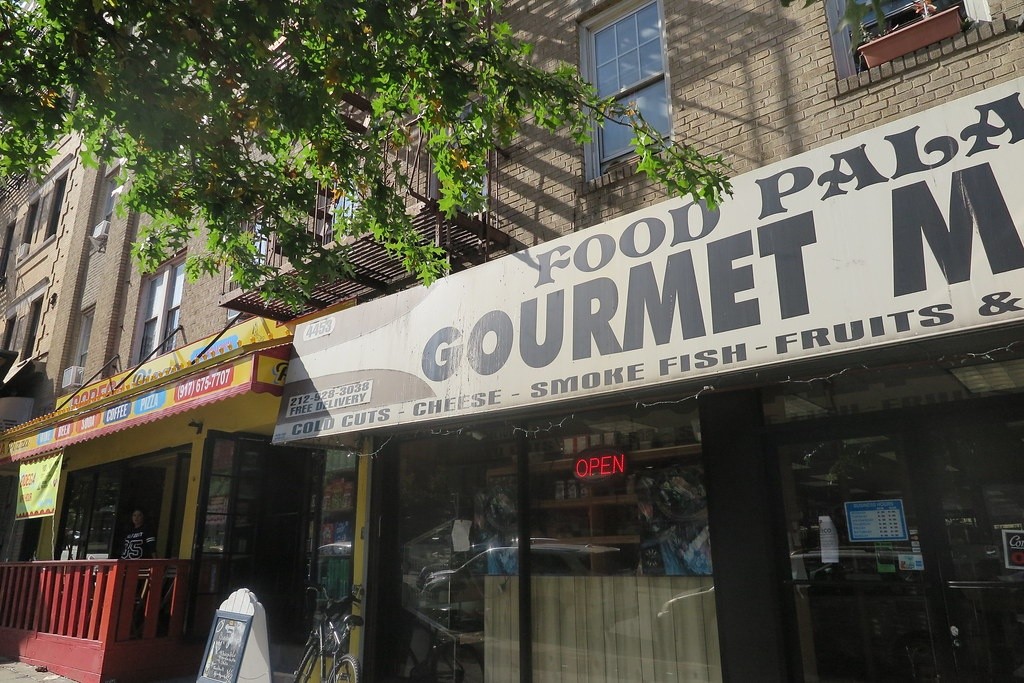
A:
[482,443,706,577]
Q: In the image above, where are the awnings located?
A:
[0,334,293,466]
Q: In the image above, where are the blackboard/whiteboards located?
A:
[196,608,254,682]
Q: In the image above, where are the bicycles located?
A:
[292,584,364,683]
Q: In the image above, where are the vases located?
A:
[856,6,962,68]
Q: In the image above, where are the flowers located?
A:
[859,0,938,44]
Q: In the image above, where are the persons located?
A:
[121,506,157,559]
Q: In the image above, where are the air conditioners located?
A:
[93,220,111,241]
[62,366,85,389]
[18,242,31,260]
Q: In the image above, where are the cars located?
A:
[420,544,621,628]
[65,530,81,546]
[416,543,516,590]
[315,542,353,606]
[509,537,560,545]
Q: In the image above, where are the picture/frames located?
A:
[195,609,253,683]
